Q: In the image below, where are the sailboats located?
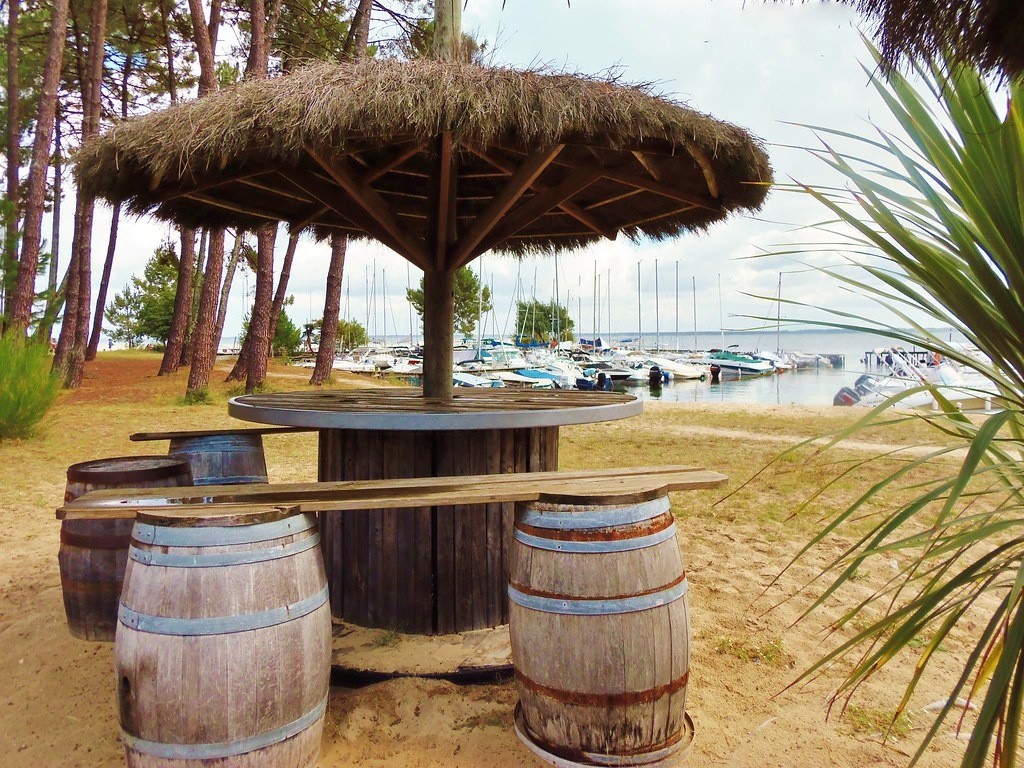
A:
[214,246,847,391]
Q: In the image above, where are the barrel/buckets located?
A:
[168,434,269,486]
[513,490,694,767]
[57,455,197,642]
[113,508,334,767]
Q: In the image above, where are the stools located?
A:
[58,456,193,641]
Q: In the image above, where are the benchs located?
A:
[62,465,728,768]
[131,429,322,487]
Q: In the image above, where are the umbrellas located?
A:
[75,0,774,401]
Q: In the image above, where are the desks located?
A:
[227,386,642,635]
